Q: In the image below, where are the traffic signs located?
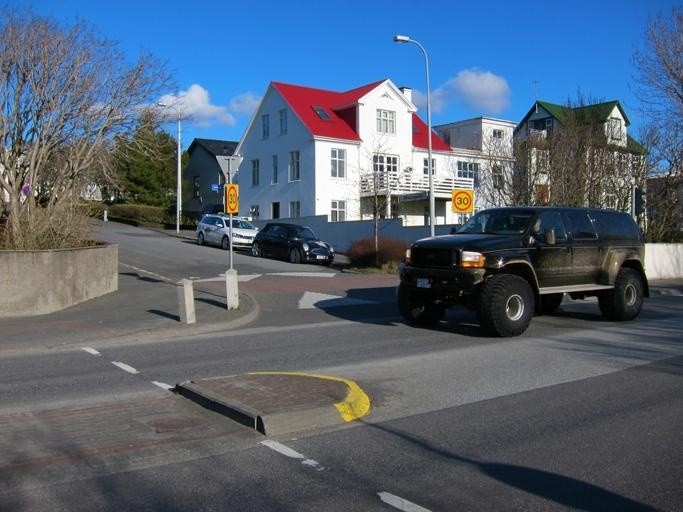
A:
[224,184,238,215]
[452,189,473,213]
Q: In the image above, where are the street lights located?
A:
[393,34,437,236]
[156,103,181,233]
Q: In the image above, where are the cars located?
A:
[250,222,334,266]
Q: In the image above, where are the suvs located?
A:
[394,203,650,337]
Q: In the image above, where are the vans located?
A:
[196,213,258,251]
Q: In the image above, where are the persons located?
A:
[518,217,547,247]
